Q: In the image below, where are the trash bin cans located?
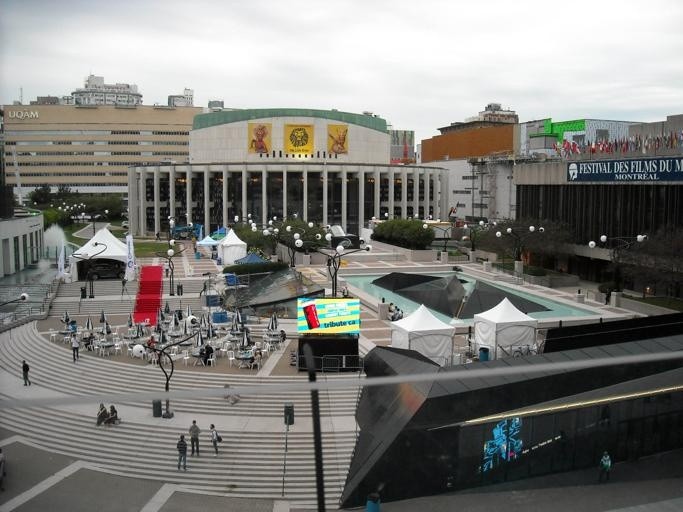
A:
[195,252,200,259]
[479,347,489,362]
[153,399,162,417]
[81,287,86,299]
[177,284,183,296]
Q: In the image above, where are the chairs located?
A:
[49,317,280,371]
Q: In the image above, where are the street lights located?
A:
[276,399,295,496]
[461,218,497,250]
[231,212,450,297]
[0,199,109,307]
[131,315,202,418]
[587,233,648,293]
[496,224,545,260]
[157,239,188,296]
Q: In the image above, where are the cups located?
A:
[303,304,320,330]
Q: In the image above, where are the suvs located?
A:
[121,221,128,228]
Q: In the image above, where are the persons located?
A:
[121,276,128,298]
[175,435,187,471]
[342,286,348,298]
[208,423,218,457]
[596,450,611,481]
[603,288,610,305]
[95,403,107,425]
[203,344,212,364]
[0,448,5,490]
[22,360,30,387]
[188,420,201,457]
[198,280,205,299]
[389,302,403,321]
[70,334,79,363]
[104,405,118,425]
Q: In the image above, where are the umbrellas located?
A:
[84,299,278,350]
